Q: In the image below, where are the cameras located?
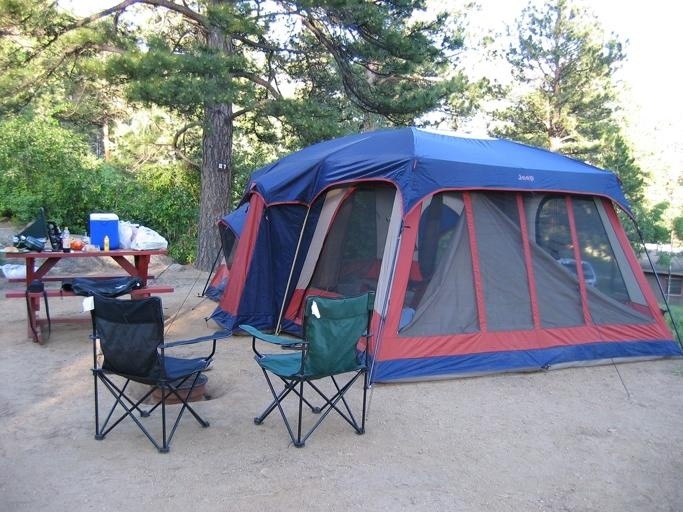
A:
[29,277,44,291]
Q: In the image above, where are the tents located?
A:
[199,125,683,394]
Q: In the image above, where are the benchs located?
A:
[4,274,173,338]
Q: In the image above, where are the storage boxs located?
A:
[90,213,119,250]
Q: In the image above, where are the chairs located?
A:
[89,290,233,453]
[239,290,375,448]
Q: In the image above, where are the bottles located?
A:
[61,226,71,253]
[103,236,110,253]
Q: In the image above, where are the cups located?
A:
[24,236,45,252]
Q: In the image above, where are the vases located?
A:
[150,374,208,404]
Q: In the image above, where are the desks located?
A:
[5,246,169,338]
